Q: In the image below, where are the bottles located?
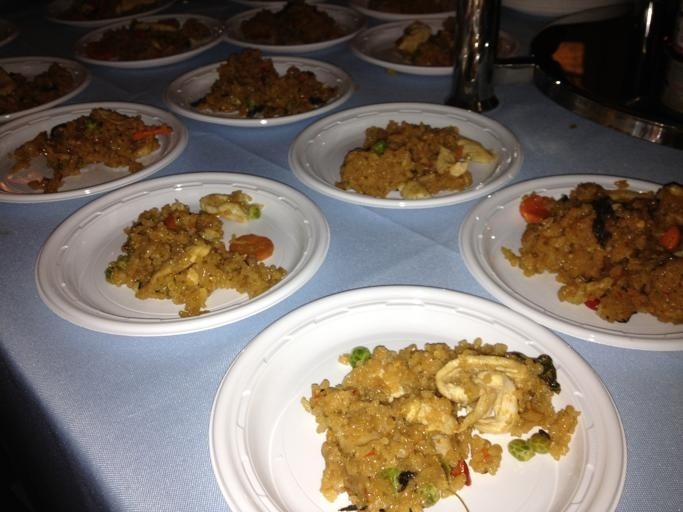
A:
[596,1,681,118]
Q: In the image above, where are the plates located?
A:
[76,15,221,70]
[452,172,682,352]
[0,54,92,122]
[354,2,458,23]
[37,0,174,26]
[288,102,523,208]
[348,20,525,78]
[0,102,189,205]
[165,56,354,129]
[221,6,372,54]
[29,170,334,341]
[210,283,631,512]
